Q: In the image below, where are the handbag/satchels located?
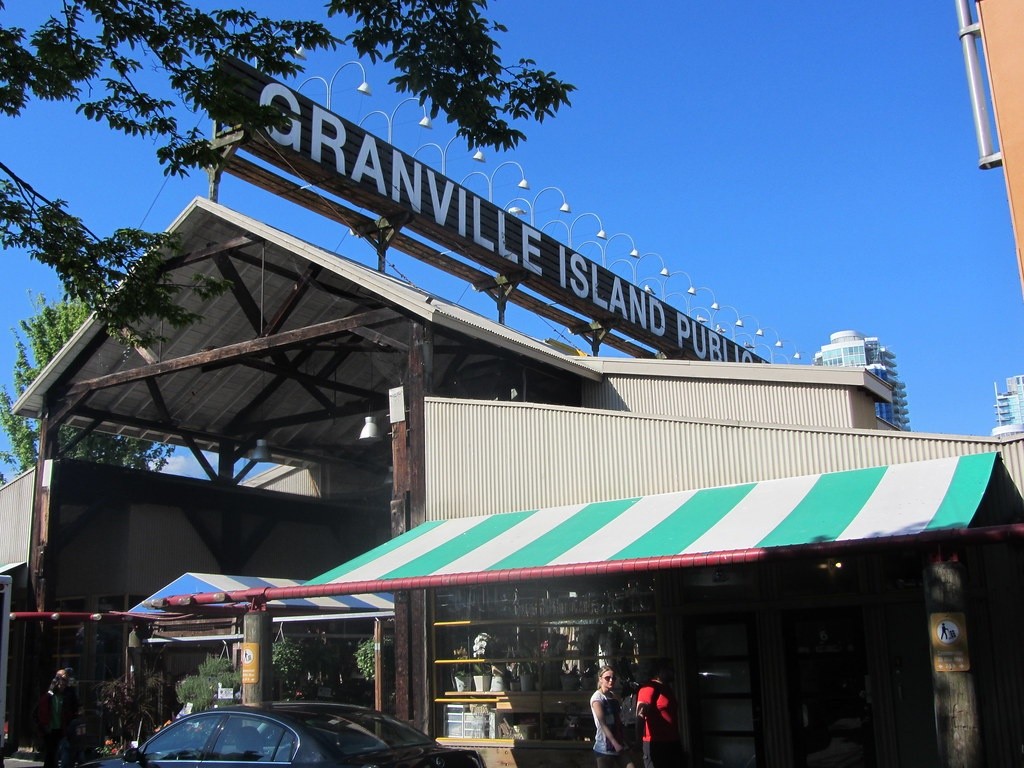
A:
[32,703,40,724]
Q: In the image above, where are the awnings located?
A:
[302,451,1024,613]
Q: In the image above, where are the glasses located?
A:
[602,676,617,682]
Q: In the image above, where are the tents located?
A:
[128,572,395,608]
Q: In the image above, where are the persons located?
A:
[636,657,687,768]
[38,667,87,768]
[590,666,635,768]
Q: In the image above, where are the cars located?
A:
[75,703,485,768]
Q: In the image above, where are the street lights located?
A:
[294,60,802,366]
